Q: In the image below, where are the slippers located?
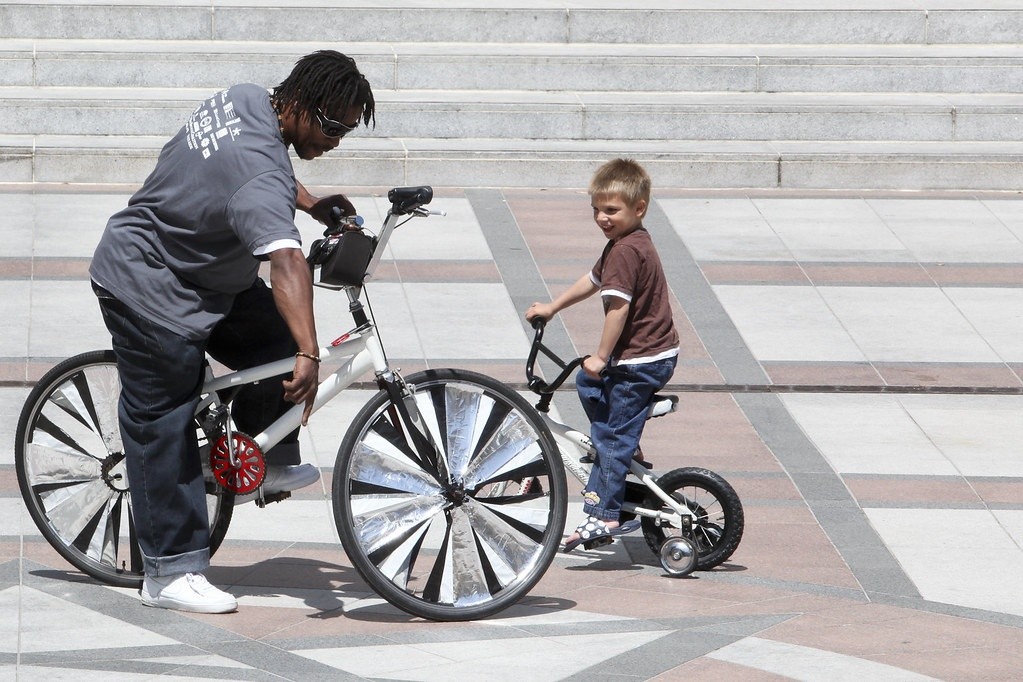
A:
[579,442,653,470]
[563,517,641,553]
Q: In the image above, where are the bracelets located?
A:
[295,352,321,362]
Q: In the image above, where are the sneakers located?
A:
[141,572,238,615]
[233,464,320,506]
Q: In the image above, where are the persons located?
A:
[89,51,375,615]
[522,158,681,555]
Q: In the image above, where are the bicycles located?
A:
[475,315,744,577]
[14,186,567,622]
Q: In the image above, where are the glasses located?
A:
[314,109,355,140]
